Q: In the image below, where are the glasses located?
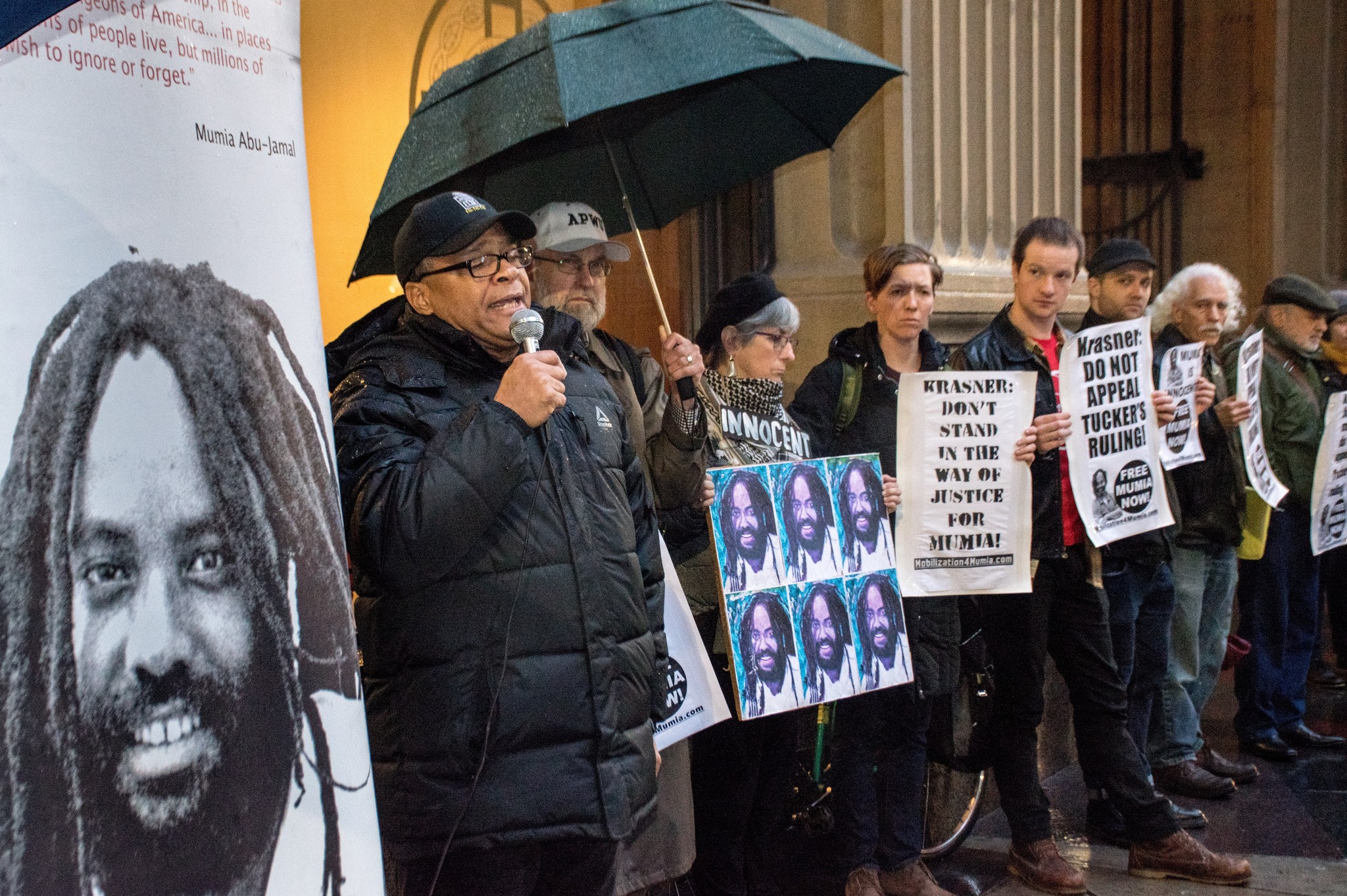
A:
[534,255,613,278]
[417,246,534,281]
[754,332,798,352]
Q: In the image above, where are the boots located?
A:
[1128,827,1253,885]
[1007,835,1087,895]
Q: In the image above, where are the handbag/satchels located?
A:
[927,592,992,774]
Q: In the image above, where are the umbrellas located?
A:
[349,0,913,410]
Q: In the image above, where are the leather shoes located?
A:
[1308,667,1344,689]
[1283,726,1347,749]
[1197,744,1260,782]
[1091,792,1210,845]
[1159,761,1240,797]
[1240,732,1293,759]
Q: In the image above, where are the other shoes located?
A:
[845,861,957,896]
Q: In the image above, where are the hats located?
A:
[530,201,632,263]
[703,271,784,335]
[393,190,538,284]
[1327,288,1347,321]
[1263,273,1338,314]
[1085,239,1159,274]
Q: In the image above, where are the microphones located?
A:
[509,309,552,443]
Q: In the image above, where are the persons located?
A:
[855,572,914,693]
[943,215,1256,894]
[781,462,842,587]
[686,271,901,896]
[1141,254,1269,805]
[1237,276,1347,763]
[799,579,862,709]
[738,593,803,720]
[786,241,1038,896]
[1300,293,1347,728]
[1072,234,1216,848]
[838,455,899,574]
[521,198,710,896]
[328,191,670,896]
[0,258,384,896]
[718,470,786,595]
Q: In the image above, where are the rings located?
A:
[686,354,693,365]
